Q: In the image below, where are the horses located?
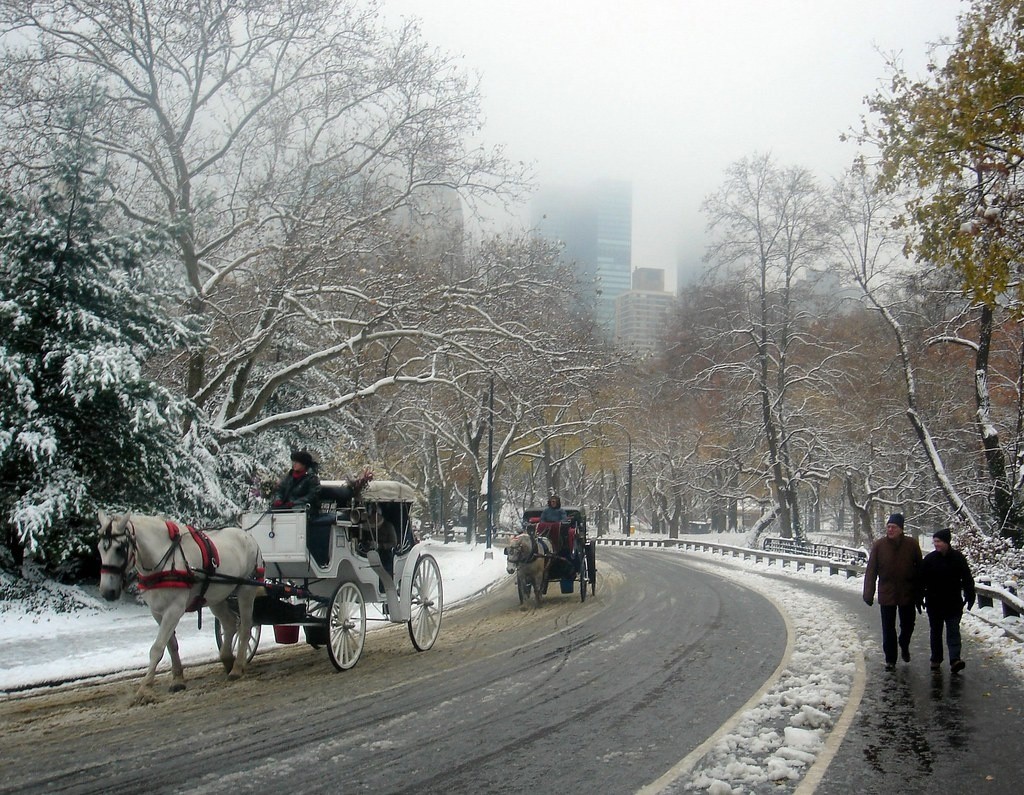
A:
[506,533,553,603]
[98,506,265,706]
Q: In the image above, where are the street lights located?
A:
[484,372,511,560]
[614,422,633,537]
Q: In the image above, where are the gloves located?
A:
[274,500,283,507]
[865,598,873,606]
[916,597,925,614]
[963,593,976,611]
[286,501,295,507]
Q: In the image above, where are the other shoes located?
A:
[899,643,910,662]
[951,661,965,673]
[930,660,940,670]
[885,662,895,670]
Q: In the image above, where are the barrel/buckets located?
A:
[305,618,326,645]
[273,626,299,644]
[560,581,574,594]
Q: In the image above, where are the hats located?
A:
[887,513,904,530]
[291,452,313,468]
[368,504,382,515]
[933,528,951,544]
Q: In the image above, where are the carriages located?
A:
[504,506,598,605]
[97,481,444,708]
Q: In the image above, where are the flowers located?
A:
[249,470,283,500]
[341,466,375,507]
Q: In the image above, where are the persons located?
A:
[541,496,566,522]
[360,506,397,594]
[274,451,321,522]
[863,514,922,671]
[923,528,976,672]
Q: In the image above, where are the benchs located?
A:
[523,517,574,554]
[307,485,351,527]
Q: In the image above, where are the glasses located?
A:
[932,540,941,545]
